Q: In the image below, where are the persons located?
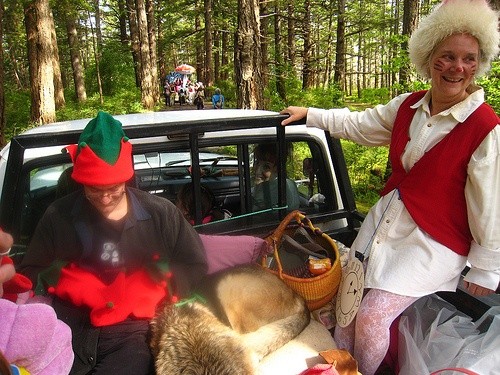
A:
[279,0,500,375]
[172,181,220,228]
[245,139,294,214]
[24,111,210,375]
[160,70,227,111]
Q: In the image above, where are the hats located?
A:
[62,111,134,186]
[409,0,499,76]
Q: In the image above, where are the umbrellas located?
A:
[174,64,197,87]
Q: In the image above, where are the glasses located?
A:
[82,183,126,198]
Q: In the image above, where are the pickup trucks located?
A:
[0,108,498,375]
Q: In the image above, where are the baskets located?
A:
[262,210,342,311]
